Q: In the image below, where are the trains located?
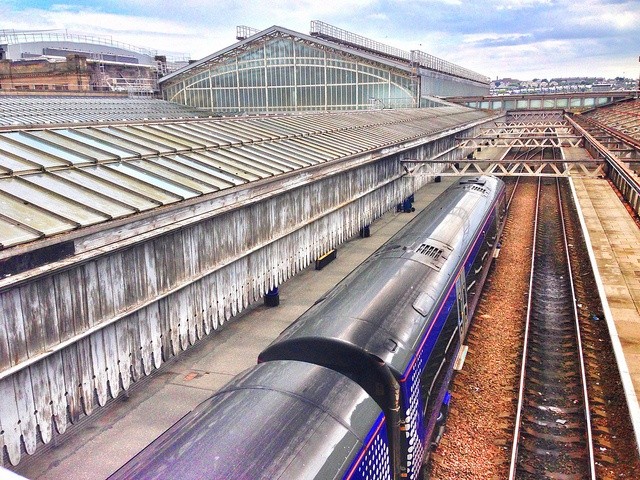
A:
[108,175,506,479]
